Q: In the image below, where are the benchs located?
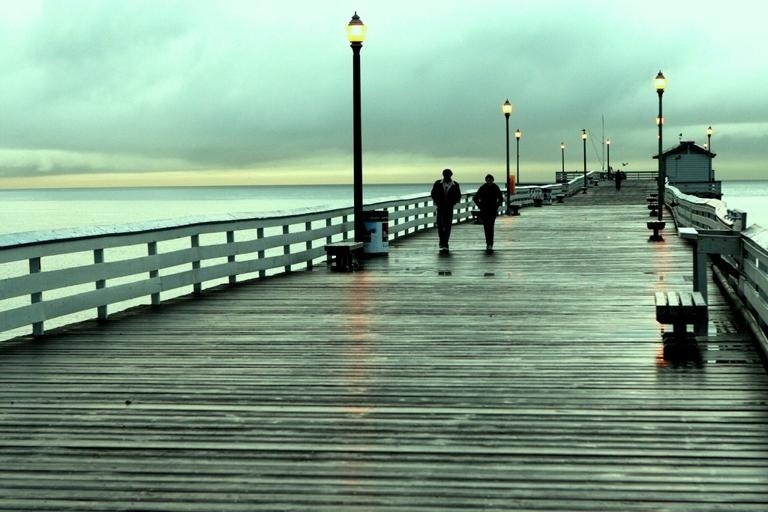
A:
[556,193,565,203]
[581,187,587,194]
[647,220,665,239]
[594,180,599,186]
[647,193,658,216]
[509,204,522,216]
[471,210,481,224]
[325,241,364,271]
[655,292,708,343]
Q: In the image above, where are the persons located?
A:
[614,170,622,191]
[472,173,504,254]
[431,169,462,254]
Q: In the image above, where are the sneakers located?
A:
[437,245,450,257]
[484,246,495,255]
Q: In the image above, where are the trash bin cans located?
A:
[542,188,552,205]
[362,210,389,257]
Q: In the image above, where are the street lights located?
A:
[345,11,367,264]
[515,129,521,186]
[582,129,587,187]
[704,127,712,192]
[561,142,564,173]
[653,71,667,220]
[606,139,610,180]
[503,98,512,215]
